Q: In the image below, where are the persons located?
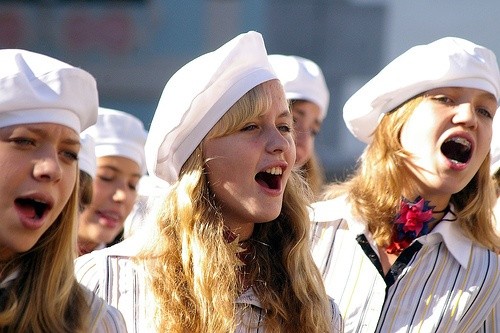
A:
[78,134,97,212]
[74,32,345,333]
[0,49,127,333]
[74,107,147,256]
[270,51,328,189]
[306,35,500,333]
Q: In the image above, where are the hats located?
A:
[268,52,330,120]
[77,108,147,182]
[1,47,98,136]
[340,36,499,145]
[142,31,277,185]
